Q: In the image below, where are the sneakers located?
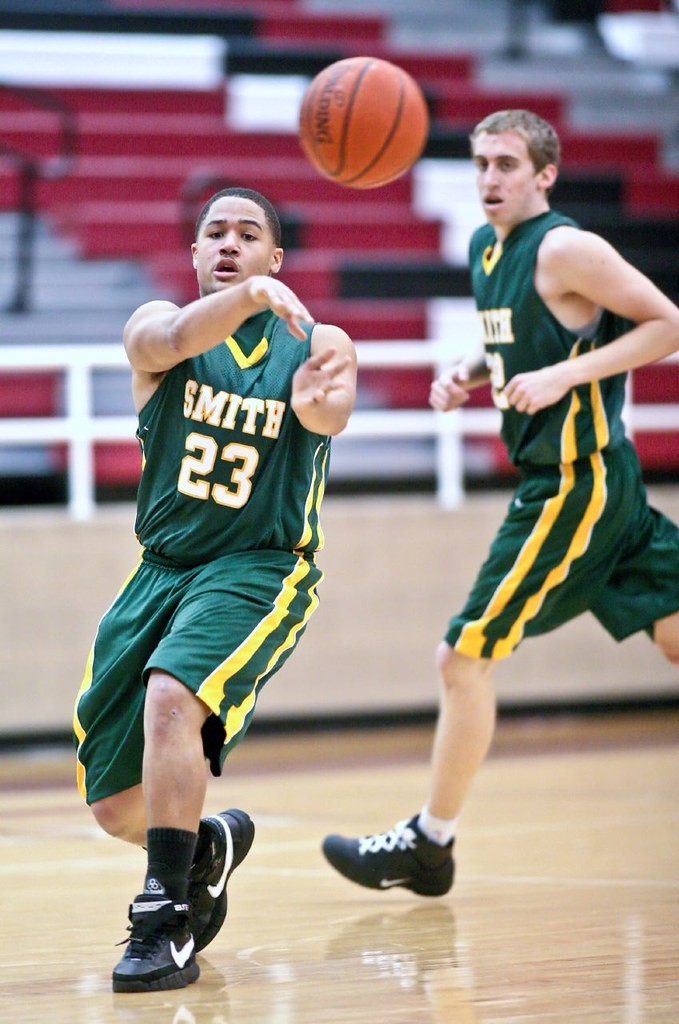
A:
[111,895,200,994]
[190,809,255,952]
[323,815,455,897]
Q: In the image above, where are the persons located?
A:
[322,109,679,896]
[71,187,358,994]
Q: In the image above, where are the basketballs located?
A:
[296,55,431,190]
